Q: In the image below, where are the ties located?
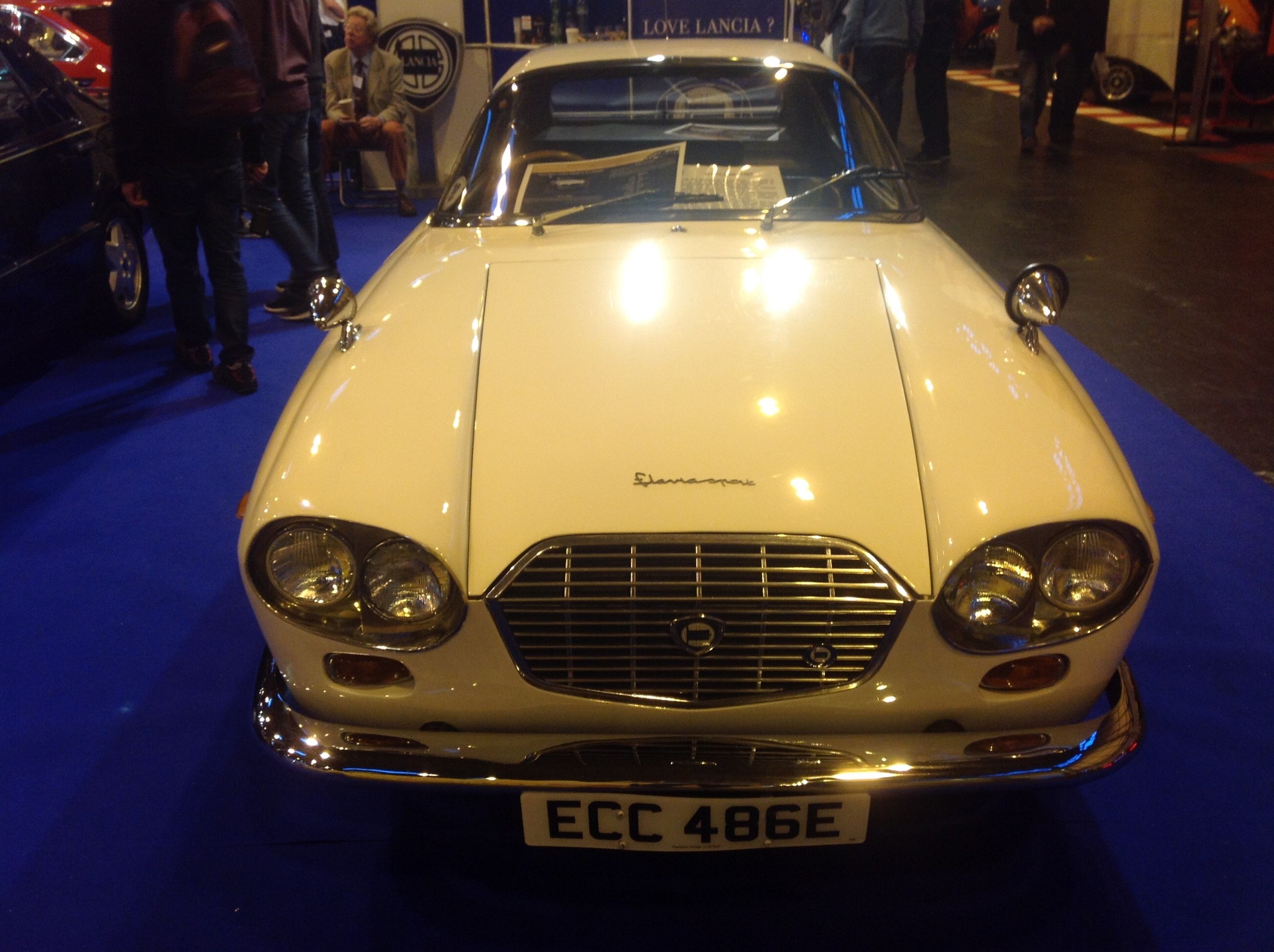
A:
[354,59,368,126]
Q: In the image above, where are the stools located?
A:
[337,144,402,214]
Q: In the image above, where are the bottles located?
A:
[512,0,628,45]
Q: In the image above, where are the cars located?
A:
[0,0,148,392]
[233,35,1158,849]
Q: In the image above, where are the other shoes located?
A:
[264,280,312,320]
[904,147,950,166]
[1021,137,1036,156]
[213,362,259,395]
[399,196,418,216]
[176,342,214,372]
[235,220,271,239]
[1047,145,1070,161]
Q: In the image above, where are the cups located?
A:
[338,98,354,120]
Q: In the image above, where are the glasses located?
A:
[342,23,368,36]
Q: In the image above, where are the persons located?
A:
[1010,1,1106,155]
[325,3,421,216]
[113,1,339,397]
[842,1,965,157]
